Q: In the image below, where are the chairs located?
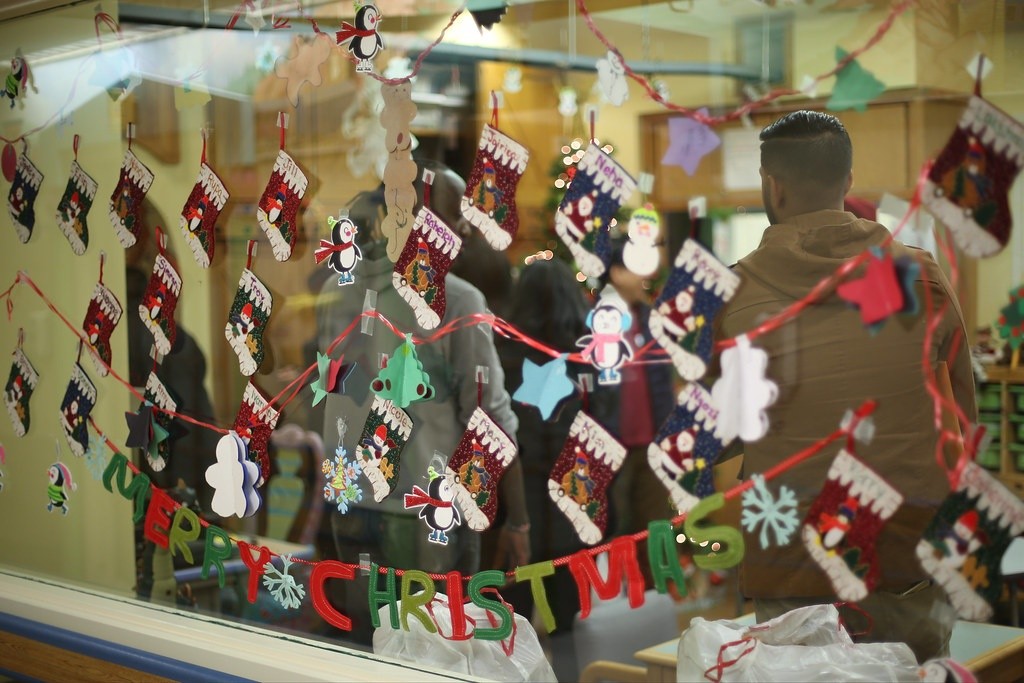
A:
[575,590,678,683]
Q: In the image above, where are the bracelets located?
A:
[506,522,530,533]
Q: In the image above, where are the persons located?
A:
[593,247,684,589]
[325,160,532,642]
[495,259,613,632]
[125,318,229,607]
[712,111,973,637]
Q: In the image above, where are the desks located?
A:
[636,611,1024,683]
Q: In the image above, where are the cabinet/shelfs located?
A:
[639,87,975,348]
[205,22,584,427]
